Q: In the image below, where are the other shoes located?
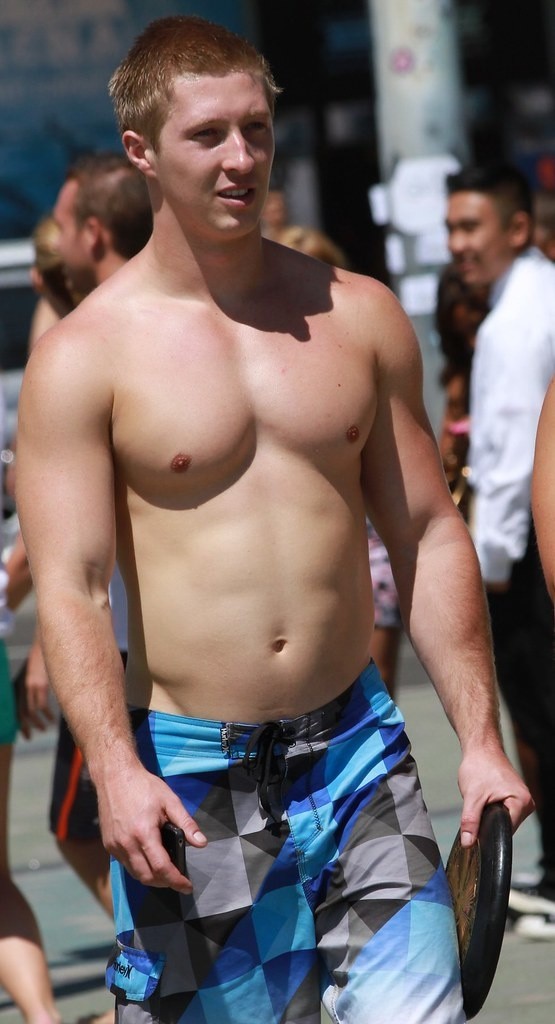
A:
[513,914,554,942]
[506,881,554,915]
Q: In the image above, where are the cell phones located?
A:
[160,822,186,878]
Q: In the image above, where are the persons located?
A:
[0,16,555,1024]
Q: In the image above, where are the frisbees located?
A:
[445,803,513,1020]
